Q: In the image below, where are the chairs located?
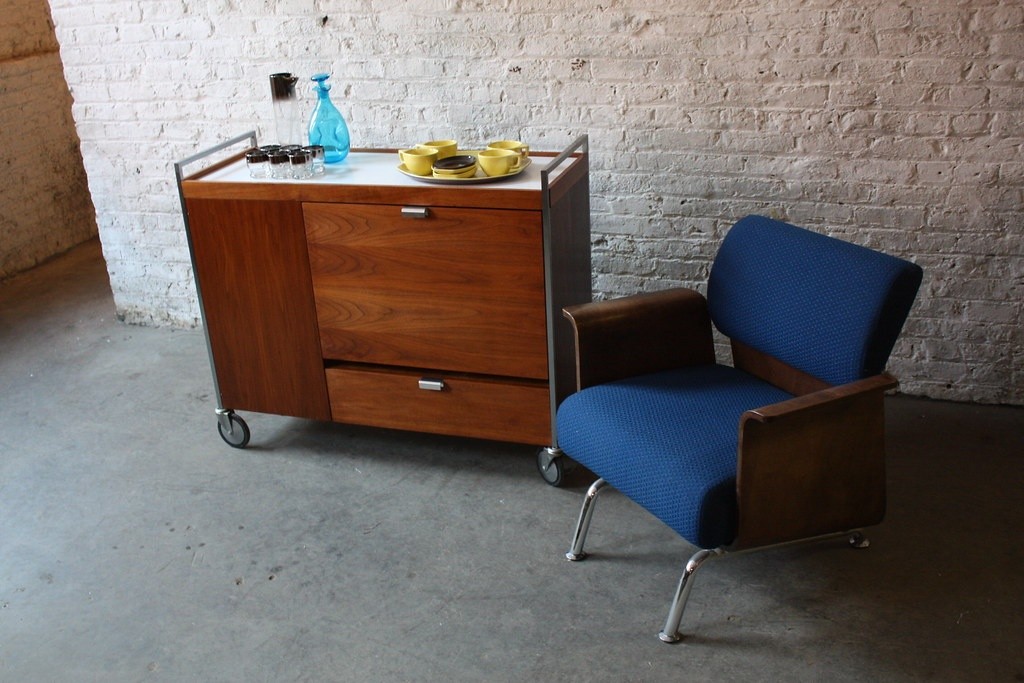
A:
[558,214,923,641]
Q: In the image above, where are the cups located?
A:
[416,140,458,160]
[478,150,522,178]
[244,145,324,181]
[398,146,439,175]
[487,140,529,164]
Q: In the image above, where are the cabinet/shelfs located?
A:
[173,131,597,486]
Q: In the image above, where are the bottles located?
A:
[307,73,350,165]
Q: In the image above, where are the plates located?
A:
[430,155,477,178]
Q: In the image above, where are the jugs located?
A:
[269,72,303,145]
